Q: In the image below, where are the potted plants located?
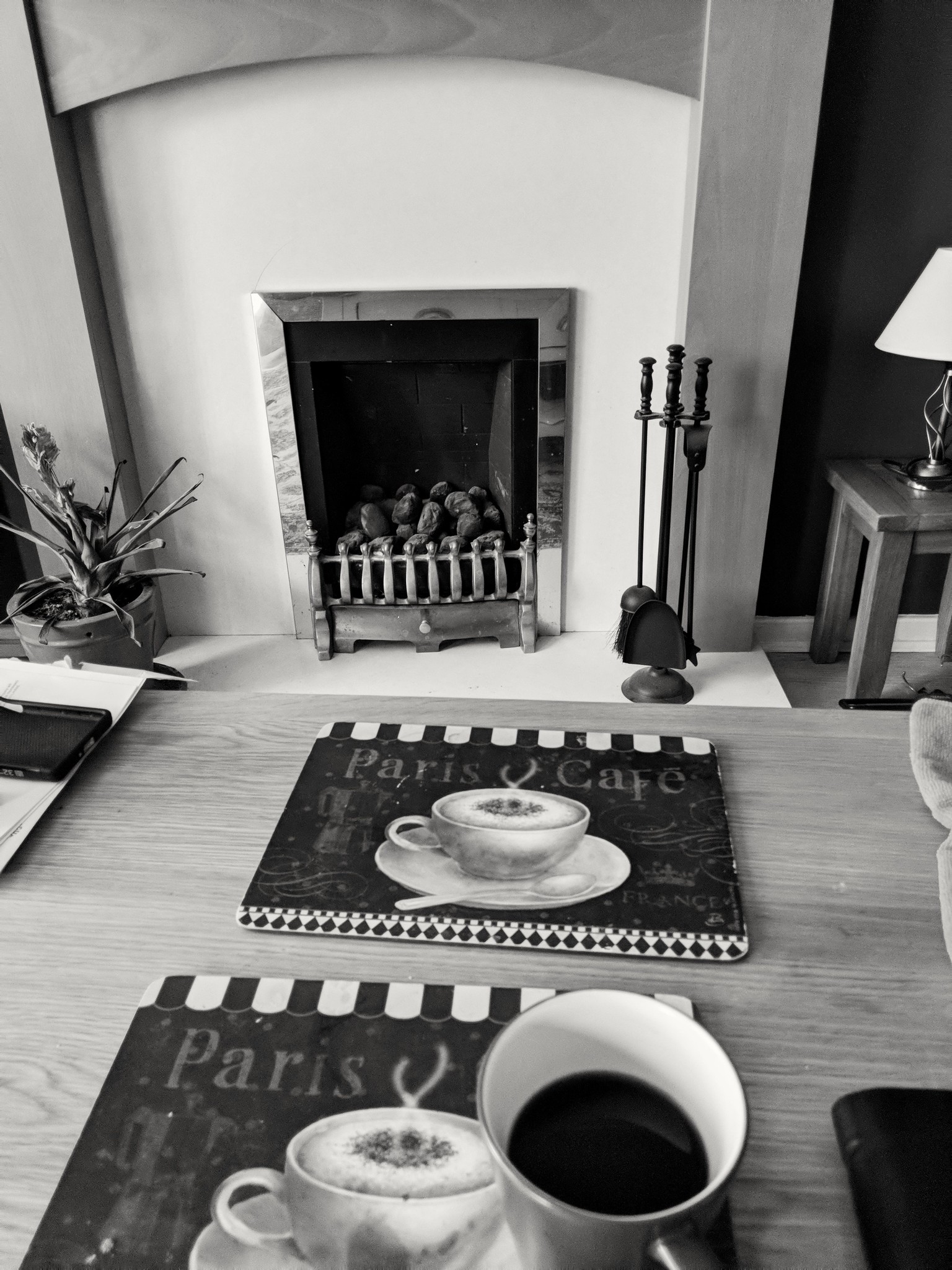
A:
[0,424,206,673]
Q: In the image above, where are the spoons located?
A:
[394,876,597,911]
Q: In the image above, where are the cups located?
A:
[382,790,591,882]
[478,989,748,1270]
[211,1104,513,1270]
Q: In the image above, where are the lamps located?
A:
[875,248,952,490]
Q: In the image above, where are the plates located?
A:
[375,823,631,910]
[188,1186,522,1270]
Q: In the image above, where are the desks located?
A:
[806,456,952,701]
